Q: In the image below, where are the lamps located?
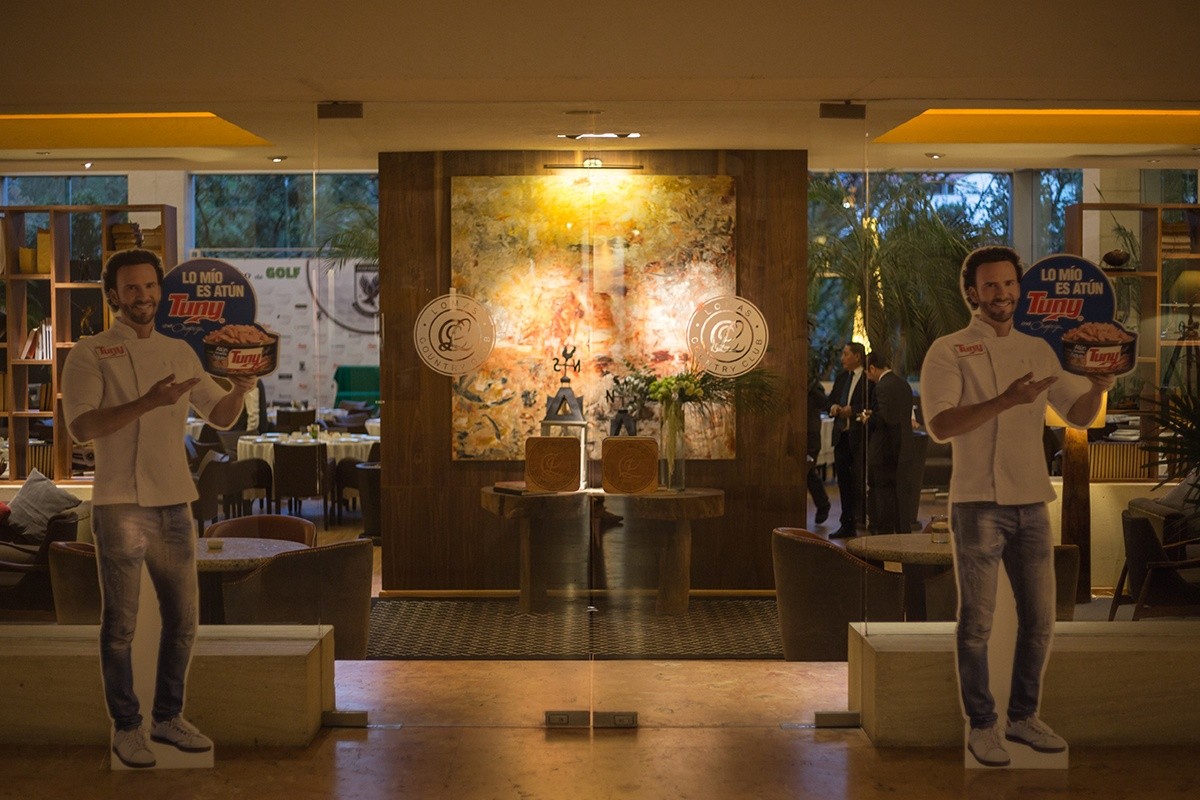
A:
[852,100,883,356]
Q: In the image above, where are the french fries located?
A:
[1061,321,1132,341]
[205,324,269,344]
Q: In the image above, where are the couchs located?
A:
[1128,497,1188,559]
[0,498,92,604]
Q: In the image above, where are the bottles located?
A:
[1103,249,1130,266]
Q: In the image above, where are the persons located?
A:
[919,245,1118,764]
[61,250,256,766]
[809,344,914,540]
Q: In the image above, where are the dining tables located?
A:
[236,434,380,532]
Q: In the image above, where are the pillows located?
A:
[8,467,81,545]
[1158,467,1200,532]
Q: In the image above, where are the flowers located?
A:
[643,361,787,424]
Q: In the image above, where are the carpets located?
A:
[357,597,783,662]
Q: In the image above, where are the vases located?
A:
[659,402,684,489]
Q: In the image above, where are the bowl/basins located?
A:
[206,537,225,550]
[202,334,281,377]
[1061,336,1136,376]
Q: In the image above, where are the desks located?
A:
[480,486,725,614]
[194,537,310,624]
[849,532,957,621]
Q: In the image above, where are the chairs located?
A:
[771,528,908,662]
[1106,510,1199,622]
[923,545,1077,623]
[50,409,382,658]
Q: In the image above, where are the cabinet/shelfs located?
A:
[1064,201,1200,602]
[0,204,178,483]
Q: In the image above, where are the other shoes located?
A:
[816,503,830,524]
[829,523,857,539]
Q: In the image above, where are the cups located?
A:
[278,424,351,443]
[930,515,949,543]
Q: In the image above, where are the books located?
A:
[0,316,53,481]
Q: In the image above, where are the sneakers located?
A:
[150,715,211,752]
[112,726,156,768]
[968,726,1010,767]
[1006,712,1066,753]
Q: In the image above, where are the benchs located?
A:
[0,623,334,751]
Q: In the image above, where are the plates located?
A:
[239,433,278,443]
